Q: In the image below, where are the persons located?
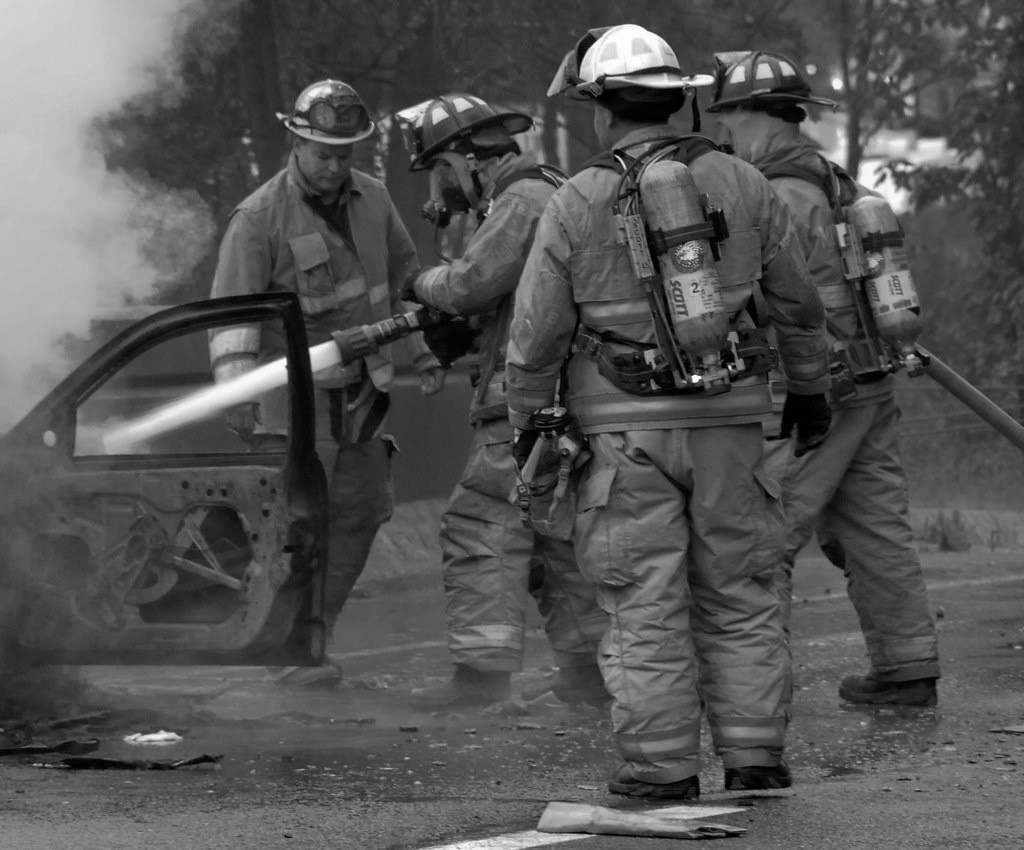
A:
[709,47,943,711]
[501,21,832,795]
[207,76,444,689]
[394,90,611,713]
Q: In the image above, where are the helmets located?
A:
[576,23,714,100]
[705,51,840,112]
[409,92,533,173]
[274,78,375,143]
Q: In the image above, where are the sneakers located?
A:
[725,760,794,798]
[838,673,938,707]
[608,764,700,802]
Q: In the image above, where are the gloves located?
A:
[780,392,832,458]
[424,319,473,365]
[511,426,539,469]
[399,266,426,304]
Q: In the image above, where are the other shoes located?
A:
[407,664,512,709]
[281,658,344,688]
[521,665,615,707]
